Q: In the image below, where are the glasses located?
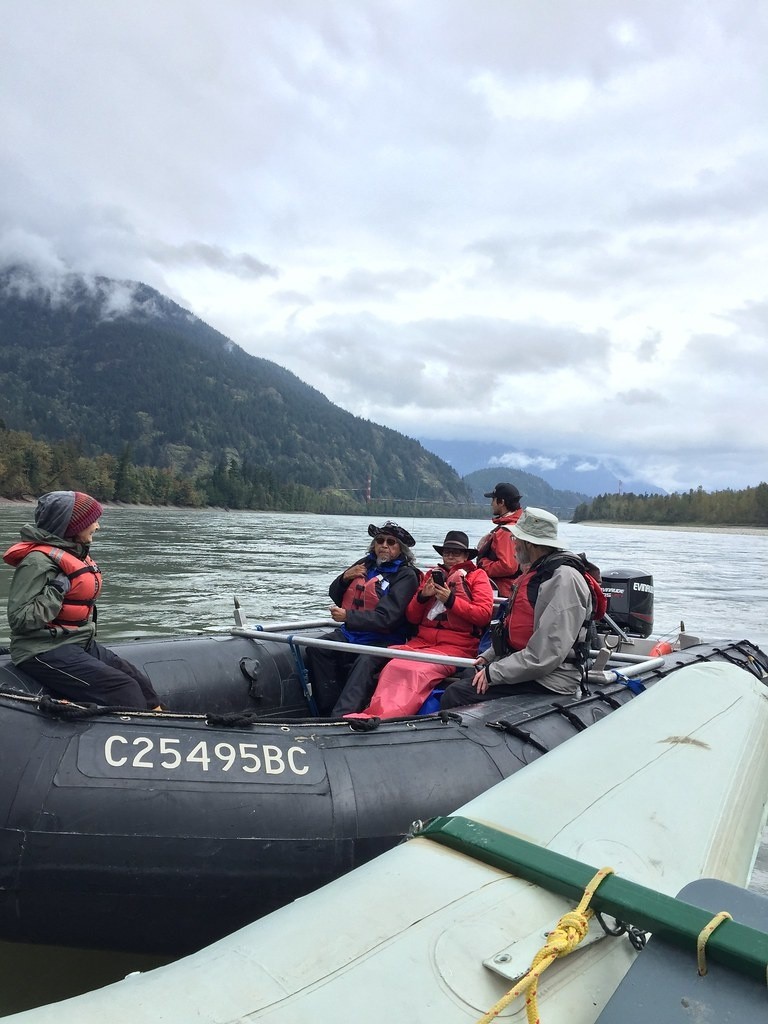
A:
[442,549,464,557]
[375,537,398,546]
[509,535,523,542]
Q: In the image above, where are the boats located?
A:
[0,564,767,951]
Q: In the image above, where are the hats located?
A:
[368,520,415,547]
[484,483,521,503]
[501,506,569,550]
[64,492,103,537]
[433,531,478,561]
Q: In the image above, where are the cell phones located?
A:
[431,570,445,589]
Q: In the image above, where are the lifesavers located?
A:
[649,641,672,658]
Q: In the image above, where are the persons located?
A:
[343,531,494,719]
[476,483,524,598]
[2,490,163,712]
[440,506,594,710]
[306,522,423,717]
